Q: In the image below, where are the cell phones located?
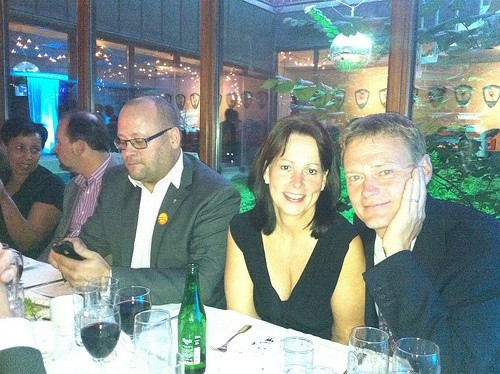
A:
[51,240,85,261]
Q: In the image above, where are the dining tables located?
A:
[0,245,414,374]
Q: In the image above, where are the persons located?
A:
[48,96,241,307]
[340,112,500,374]
[35,110,123,264]
[0,117,66,260]
[224,115,366,347]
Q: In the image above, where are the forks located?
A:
[208,324,253,352]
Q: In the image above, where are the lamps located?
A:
[324,0,377,74]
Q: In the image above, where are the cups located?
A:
[393,336,442,374]
[345,326,390,373]
[0,242,175,374]
[278,337,315,374]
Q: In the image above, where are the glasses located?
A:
[114,127,172,150]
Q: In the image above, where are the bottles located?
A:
[178,263,207,374]
